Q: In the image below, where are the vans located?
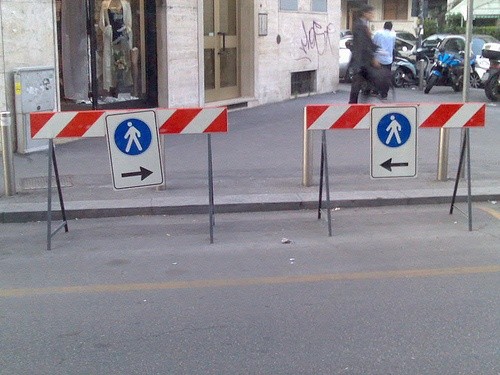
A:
[339,32,418,82]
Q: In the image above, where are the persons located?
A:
[349,4,390,103]
[370,21,397,100]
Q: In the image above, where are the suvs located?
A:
[434,34,500,88]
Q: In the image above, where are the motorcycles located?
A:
[481,43,500,103]
[424,47,476,94]
[390,39,440,88]
